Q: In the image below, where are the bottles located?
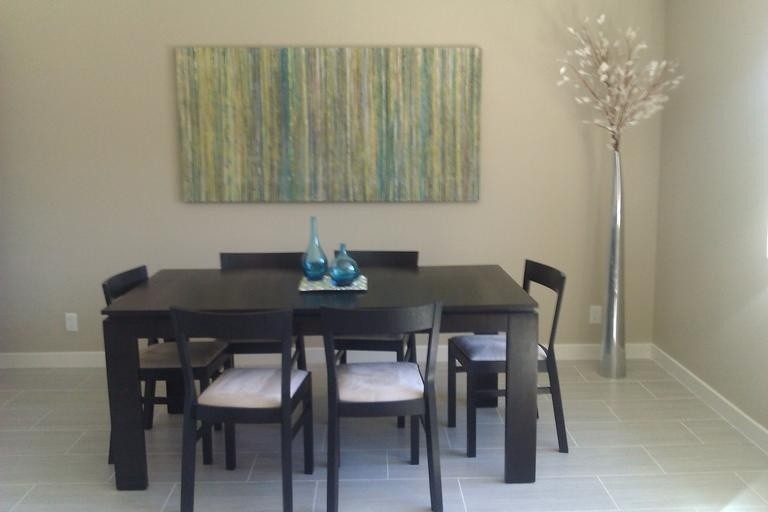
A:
[329,243,361,288]
[300,215,327,279]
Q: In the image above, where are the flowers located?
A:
[557,9,687,154]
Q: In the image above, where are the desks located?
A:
[102,265,541,490]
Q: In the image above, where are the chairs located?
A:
[446,258,569,458]
[318,303,446,511]
[100,264,233,465]
[219,251,310,372]
[331,248,420,428]
[167,303,316,512]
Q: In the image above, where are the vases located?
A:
[594,149,631,380]
